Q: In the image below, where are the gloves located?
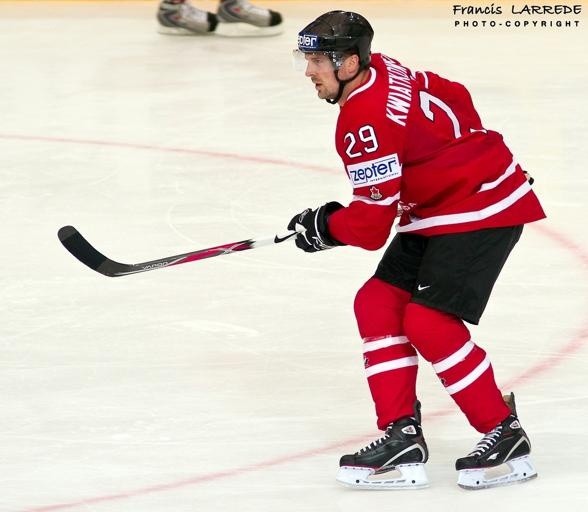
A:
[285,200,346,254]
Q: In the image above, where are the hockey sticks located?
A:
[58,203,403,277]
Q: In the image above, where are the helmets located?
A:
[297,10,374,74]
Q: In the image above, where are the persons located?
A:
[278,9,547,475]
[156,0,283,34]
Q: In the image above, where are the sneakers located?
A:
[156,1,220,36]
[216,1,287,30]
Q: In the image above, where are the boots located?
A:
[455,392,533,473]
[337,400,430,473]
